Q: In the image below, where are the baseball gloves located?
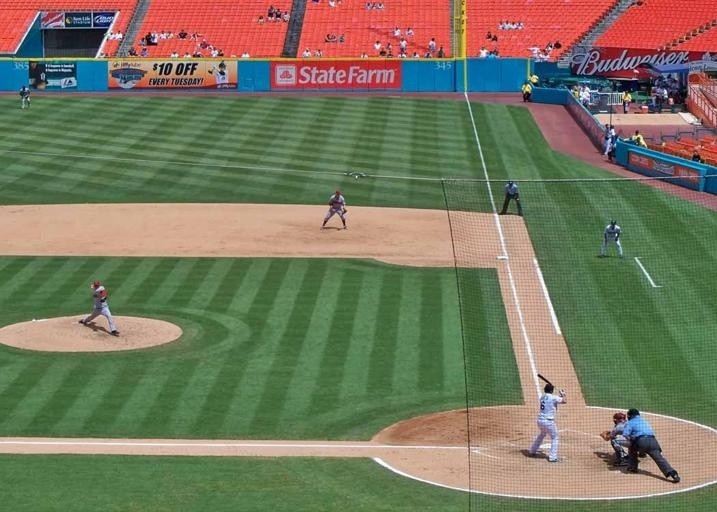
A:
[600,431,611,441]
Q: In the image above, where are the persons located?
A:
[478,20,526,58]
[600,412,646,466]
[19,86,31,109]
[99,29,224,60]
[302,48,322,57]
[527,39,561,62]
[692,150,701,162]
[572,84,590,110]
[528,384,567,462]
[654,75,688,114]
[257,4,291,24]
[623,88,632,114]
[321,191,348,230]
[78,281,120,336]
[600,220,623,258]
[324,32,345,42]
[522,73,539,102]
[365,1,384,10]
[498,181,523,216]
[604,124,647,163]
[360,25,445,58]
[230,51,250,58]
[623,408,680,483]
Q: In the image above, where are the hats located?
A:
[510,182,513,186]
[336,190,341,197]
[611,221,615,225]
[544,384,553,392]
[94,281,100,288]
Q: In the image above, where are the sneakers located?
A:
[671,473,679,483]
[616,458,638,473]
[112,330,119,335]
[79,319,84,323]
[528,451,543,456]
[549,457,559,462]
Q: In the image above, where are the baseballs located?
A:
[31,318,36,322]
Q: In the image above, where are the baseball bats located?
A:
[536,373,560,392]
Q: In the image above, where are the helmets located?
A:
[613,408,639,424]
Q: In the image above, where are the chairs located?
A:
[2,2,715,64]
[645,70,715,164]
